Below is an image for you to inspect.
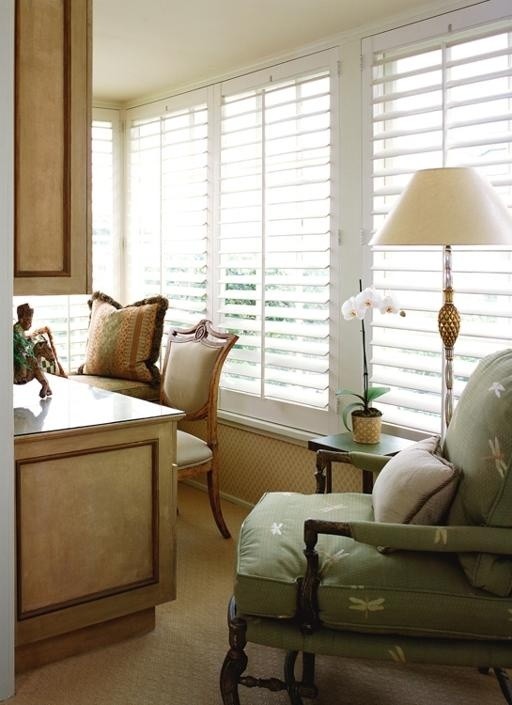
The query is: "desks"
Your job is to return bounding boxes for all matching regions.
[309,432,419,494]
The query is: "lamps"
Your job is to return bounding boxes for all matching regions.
[368,164,512,428]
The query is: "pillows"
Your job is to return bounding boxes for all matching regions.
[370,434,463,554]
[76,290,168,388]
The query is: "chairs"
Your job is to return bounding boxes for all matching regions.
[217,347,510,705]
[159,319,240,541]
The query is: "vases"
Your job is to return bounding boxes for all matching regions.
[349,411,383,445]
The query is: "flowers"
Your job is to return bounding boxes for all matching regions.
[335,277,407,436]
